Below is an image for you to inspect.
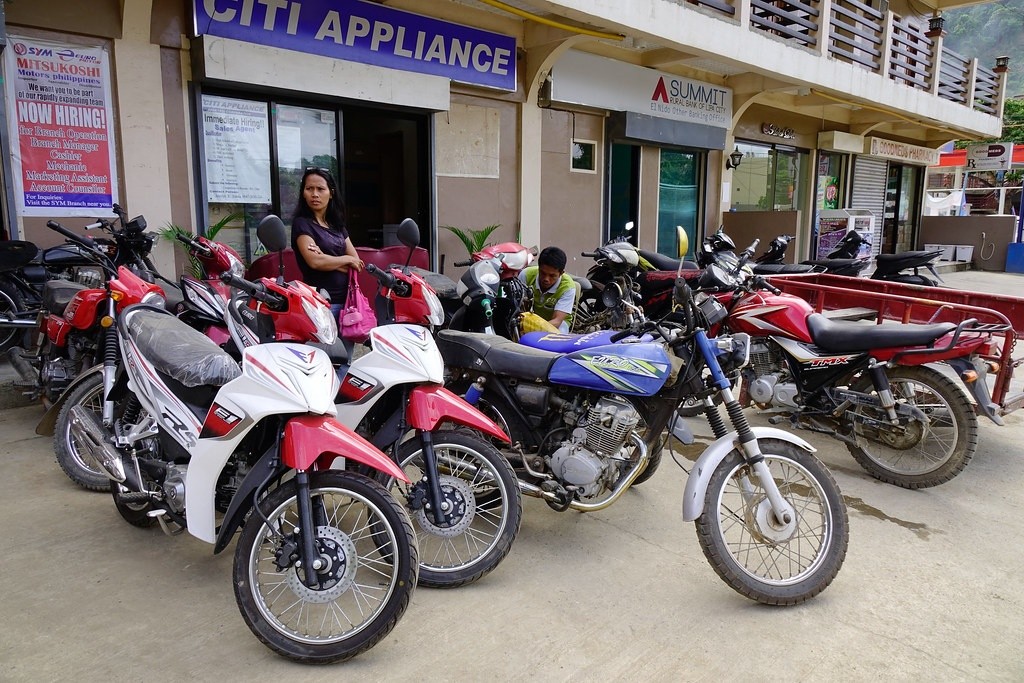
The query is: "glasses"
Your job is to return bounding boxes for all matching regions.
[305,165,332,175]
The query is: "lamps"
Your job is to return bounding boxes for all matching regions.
[726,145,744,170]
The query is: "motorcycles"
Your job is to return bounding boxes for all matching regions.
[634,228,1005,488]
[387,243,850,609]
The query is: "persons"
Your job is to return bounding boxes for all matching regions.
[514,246,576,334]
[292,167,365,400]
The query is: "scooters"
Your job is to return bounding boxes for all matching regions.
[0,202,524,666]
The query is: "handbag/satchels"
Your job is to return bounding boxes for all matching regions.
[338,266,377,342]
